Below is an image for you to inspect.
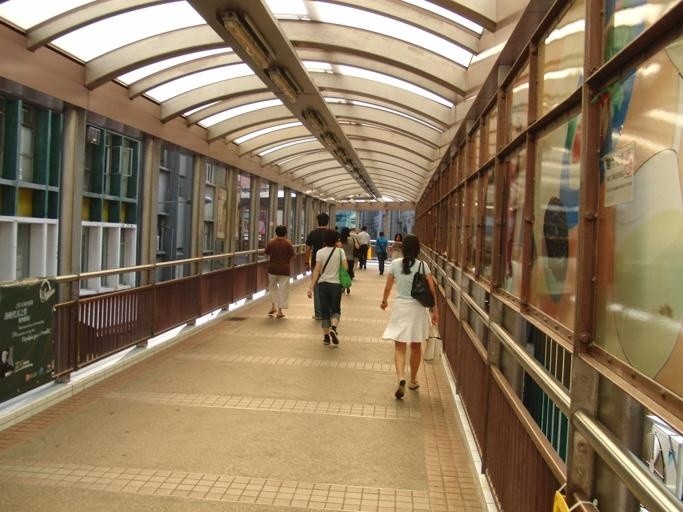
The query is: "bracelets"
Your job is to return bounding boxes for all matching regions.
[308,285,312,289]
[304,262,308,265]
[381,300,386,304]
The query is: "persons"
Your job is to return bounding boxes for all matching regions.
[379,234,439,398]
[334,226,401,294]
[265,225,295,316]
[304,212,341,321]
[306,228,348,344]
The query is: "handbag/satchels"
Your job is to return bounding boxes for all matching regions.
[410,273,436,308]
[424,337,445,366]
[384,252,388,260]
[339,268,352,288]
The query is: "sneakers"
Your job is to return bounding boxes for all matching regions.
[408,382,422,391]
[267,308,286,319]
[393,378,406,398]
[322,330,340,347]
[359,266,384,276]
[366,247,373,261]
[310,315,322,320]
[352,245,361,257]
